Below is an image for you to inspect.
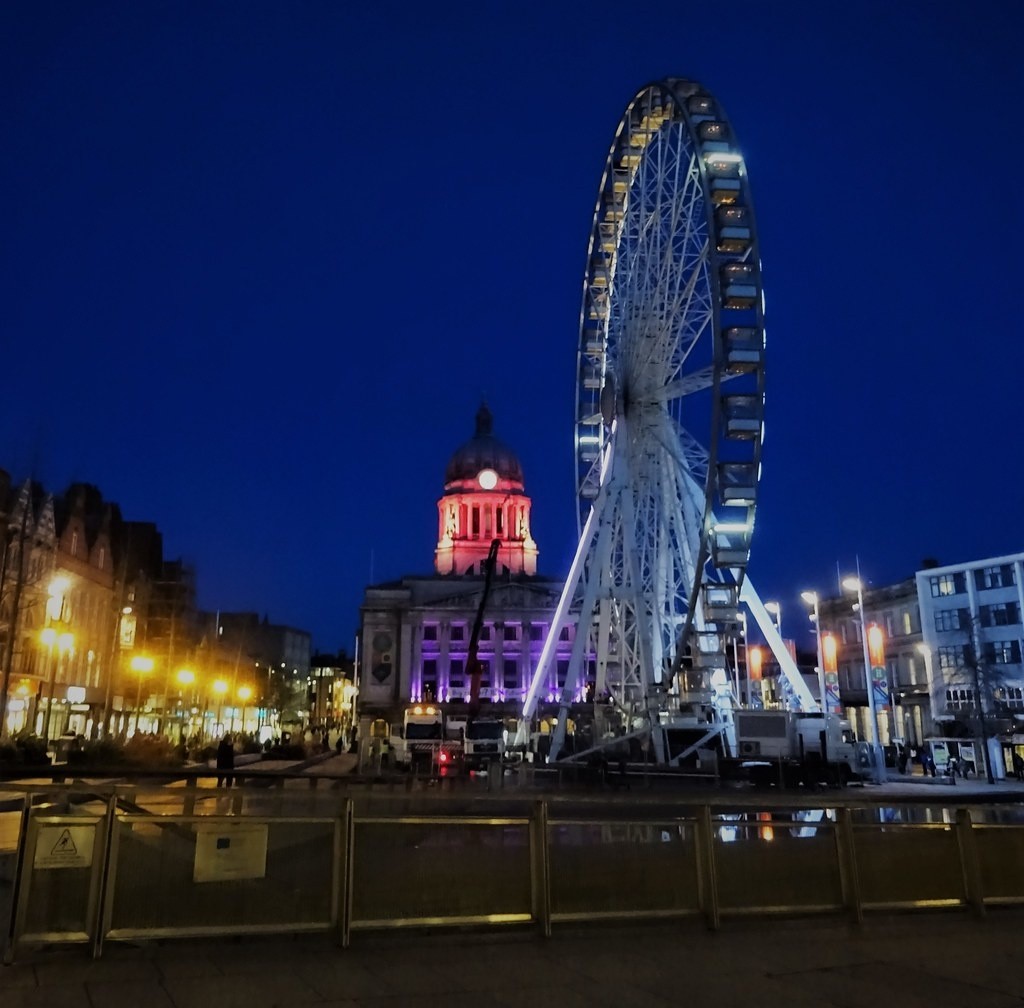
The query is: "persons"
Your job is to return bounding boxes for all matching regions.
[897,744,936,777]
[217,733,235,788]
[1013,753,1024,782]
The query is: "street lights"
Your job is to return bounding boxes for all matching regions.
[799,590,828,715]
[237,685,253,735]
[176,669,195,743]
[128,654,155,734]
[210,677,229,740]
[841,577,887,785]
[42,628,77,737]
[736,610,757,709]
[763,599,786,713]
